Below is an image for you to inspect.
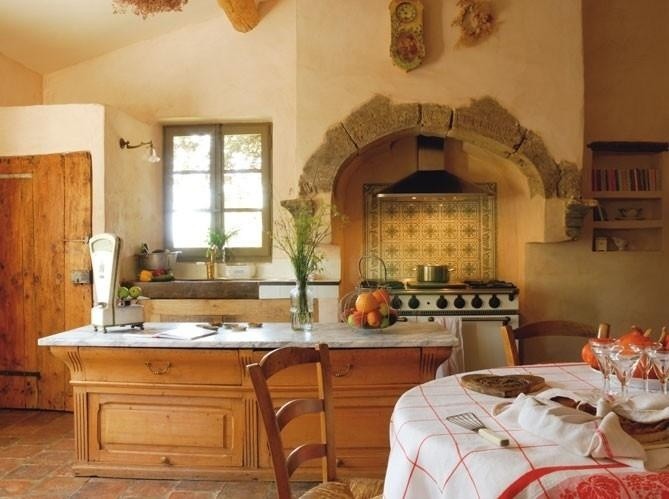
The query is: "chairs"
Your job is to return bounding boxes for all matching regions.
[499,321,611,366]
[246,342,385,499]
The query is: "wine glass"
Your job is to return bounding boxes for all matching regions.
[588,338,669,399]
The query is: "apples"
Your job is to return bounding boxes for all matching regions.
[129,285,141,297]
[118,287,128,298]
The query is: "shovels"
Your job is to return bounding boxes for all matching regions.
[445,412,509,449]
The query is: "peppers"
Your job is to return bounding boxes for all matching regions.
[139,270,153,281]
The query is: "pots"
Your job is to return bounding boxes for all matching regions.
[410,264,455,283]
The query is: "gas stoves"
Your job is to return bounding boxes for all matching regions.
[359,279,520,315]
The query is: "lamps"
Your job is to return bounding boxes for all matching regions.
[119,135,161,164]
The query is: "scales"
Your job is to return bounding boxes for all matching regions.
[87,232,149,333]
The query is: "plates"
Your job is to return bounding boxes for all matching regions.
[614,216,645,220]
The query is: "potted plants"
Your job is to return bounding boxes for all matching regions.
[267,199,345,331]
[206,226,237,263]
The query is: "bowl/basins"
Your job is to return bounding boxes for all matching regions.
[139,252,178,270]
[613,240,628,250]
[618,207,642,216]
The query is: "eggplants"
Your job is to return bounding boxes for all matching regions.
[151,267,173,276]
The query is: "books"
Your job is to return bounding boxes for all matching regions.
[151,326,216,340]
[592,168,661,192]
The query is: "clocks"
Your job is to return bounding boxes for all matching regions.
[389,0,426,73]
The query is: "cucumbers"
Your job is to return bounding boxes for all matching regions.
[155,272,176,283]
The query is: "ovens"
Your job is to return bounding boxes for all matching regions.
[455,313,520,372]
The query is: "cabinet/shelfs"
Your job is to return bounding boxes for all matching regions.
[253,346,456,482]
[585,139,666,253]
[51,347,252,480]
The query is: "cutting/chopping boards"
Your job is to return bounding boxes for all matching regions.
[461,373,545,398]
